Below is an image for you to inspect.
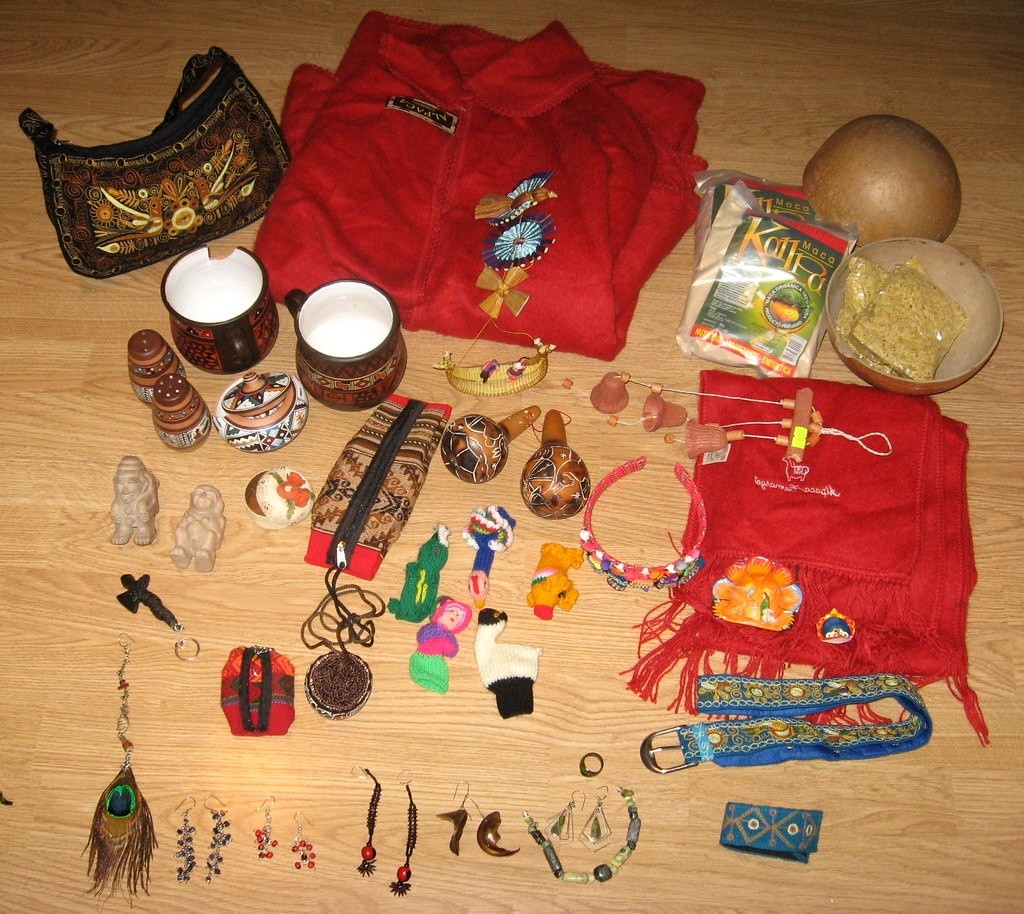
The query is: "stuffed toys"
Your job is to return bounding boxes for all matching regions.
[527,542,584,621]
[388,523,453,623]
[410,596,472,693]
[473,608,542,720]
[461,505,516,608]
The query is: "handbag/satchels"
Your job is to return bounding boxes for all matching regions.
[303,394,459,581]
[19,46,292,279]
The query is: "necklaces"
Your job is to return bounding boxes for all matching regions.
[522,787,641,883]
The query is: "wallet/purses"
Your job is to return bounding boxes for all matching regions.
[220,645,296,738]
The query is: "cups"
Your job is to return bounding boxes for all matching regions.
[161,245,279,373]
[285,278,405,412]
[244,465,315,530]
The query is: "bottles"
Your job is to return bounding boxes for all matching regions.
[128,330,186,404]
[151,376,211,450]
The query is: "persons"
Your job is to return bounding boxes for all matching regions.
[108,456,160,545]
[169,483,226,572]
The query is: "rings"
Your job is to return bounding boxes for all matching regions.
[580,753,604,778]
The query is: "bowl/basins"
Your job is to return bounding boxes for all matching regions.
[802,115,962,247]
[825,238,1003,396]
